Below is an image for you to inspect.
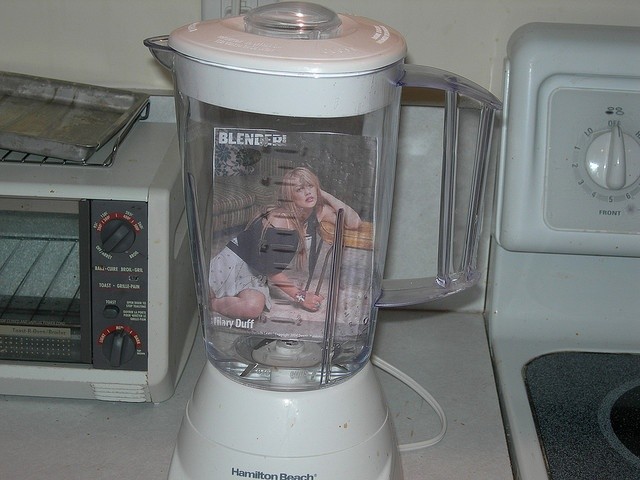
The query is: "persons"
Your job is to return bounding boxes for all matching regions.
[205,164,361,321]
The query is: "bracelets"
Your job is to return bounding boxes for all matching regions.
[295,288,307,303]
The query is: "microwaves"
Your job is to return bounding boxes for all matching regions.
[1,86,202,401]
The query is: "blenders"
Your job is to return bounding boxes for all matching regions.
[143,0,504,480]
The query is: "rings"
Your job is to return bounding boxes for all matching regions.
[317,302,320,307]
